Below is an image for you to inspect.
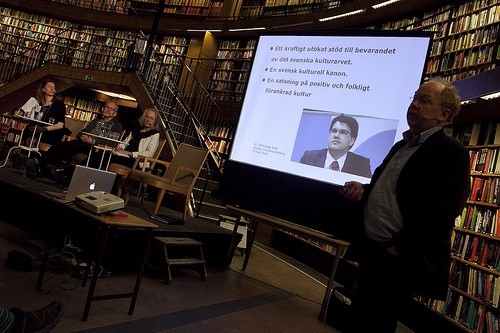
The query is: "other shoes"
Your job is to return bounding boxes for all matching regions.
[20,153,36,171]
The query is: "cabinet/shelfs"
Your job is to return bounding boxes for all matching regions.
[0,0,500,333]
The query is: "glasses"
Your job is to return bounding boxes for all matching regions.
[103,105,116,112]
[329,128,352,137]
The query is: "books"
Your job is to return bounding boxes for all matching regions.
[197,121,232,174]
[51,0,341,20]
[0,5,187,93]
[0,114,37,147]
[55,93,103,122]
[312,240,338,254]
[204,37,256,102]
[366,0,500,333]
[75,191,124,214]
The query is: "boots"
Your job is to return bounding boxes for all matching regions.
[9,300,64,333]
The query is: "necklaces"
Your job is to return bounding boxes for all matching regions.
[40,103,52,120]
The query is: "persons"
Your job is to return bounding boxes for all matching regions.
[300,115,371,178]
[0,298,65,333]
[34,101,122,178]
[19,78,66,158]
[69,108,161,178]
[340,77,471,333]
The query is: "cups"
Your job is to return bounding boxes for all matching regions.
[48,118,55,124]
[99,130,121,140]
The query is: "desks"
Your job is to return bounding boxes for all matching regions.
[37,193,159,322]
[83,132,129,171]
[0,114,54,168]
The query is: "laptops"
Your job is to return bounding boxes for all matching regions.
[44,165,116,201]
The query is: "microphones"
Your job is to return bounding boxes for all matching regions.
[423,115,438,120]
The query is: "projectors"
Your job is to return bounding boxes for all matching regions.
[74,191,125,213]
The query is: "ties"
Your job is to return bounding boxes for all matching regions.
[331,161,339,171]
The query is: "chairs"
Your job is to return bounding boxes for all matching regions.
[108,139,166,197]
[124,143,210,221]
